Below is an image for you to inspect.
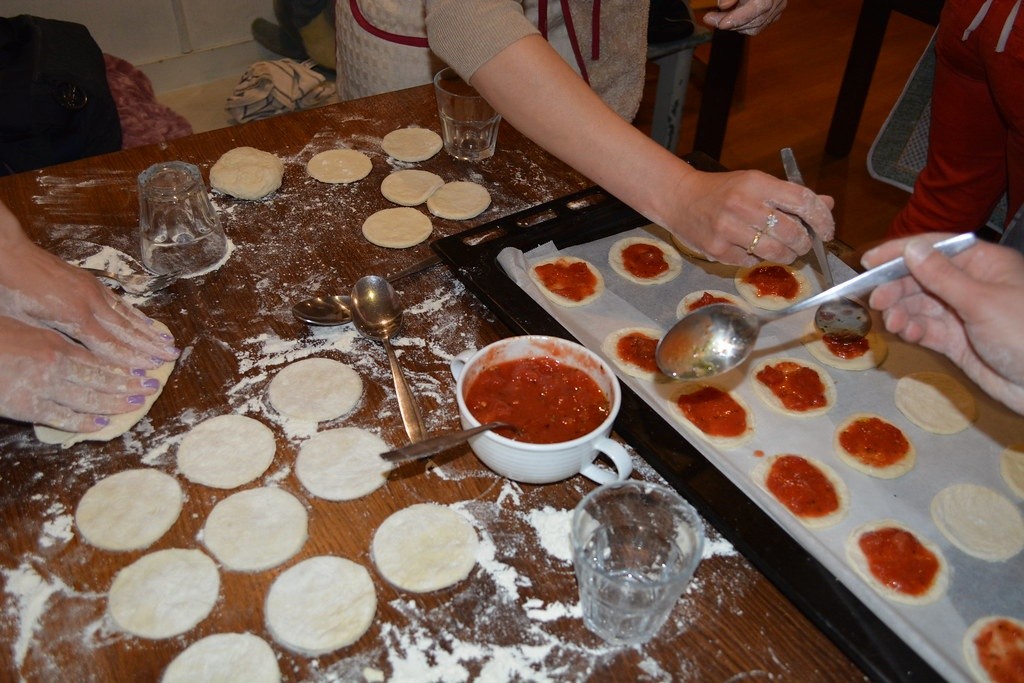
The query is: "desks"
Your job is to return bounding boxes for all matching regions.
[0,79,1024,683]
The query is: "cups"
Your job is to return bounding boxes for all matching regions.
[448,335,632,489]
[568,479,705,646]
[137,162,229,274]
[433,66,501,162]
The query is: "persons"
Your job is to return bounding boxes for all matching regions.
[335,0,835,268]
[884,0,1024,240]
[860,233,1024,416]
[0,201,182,434]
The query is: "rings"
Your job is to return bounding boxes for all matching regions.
[762,209,778,234]
[747,232,762,254]
[111,300,122,310]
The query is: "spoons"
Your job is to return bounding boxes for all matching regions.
[291,255,445,328]
[780,147,872,344]
[349,275,428,445]
[655,231,976,380]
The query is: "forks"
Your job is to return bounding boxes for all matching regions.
[85,267,185,296]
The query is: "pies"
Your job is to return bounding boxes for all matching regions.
[33,128,1024,683]
